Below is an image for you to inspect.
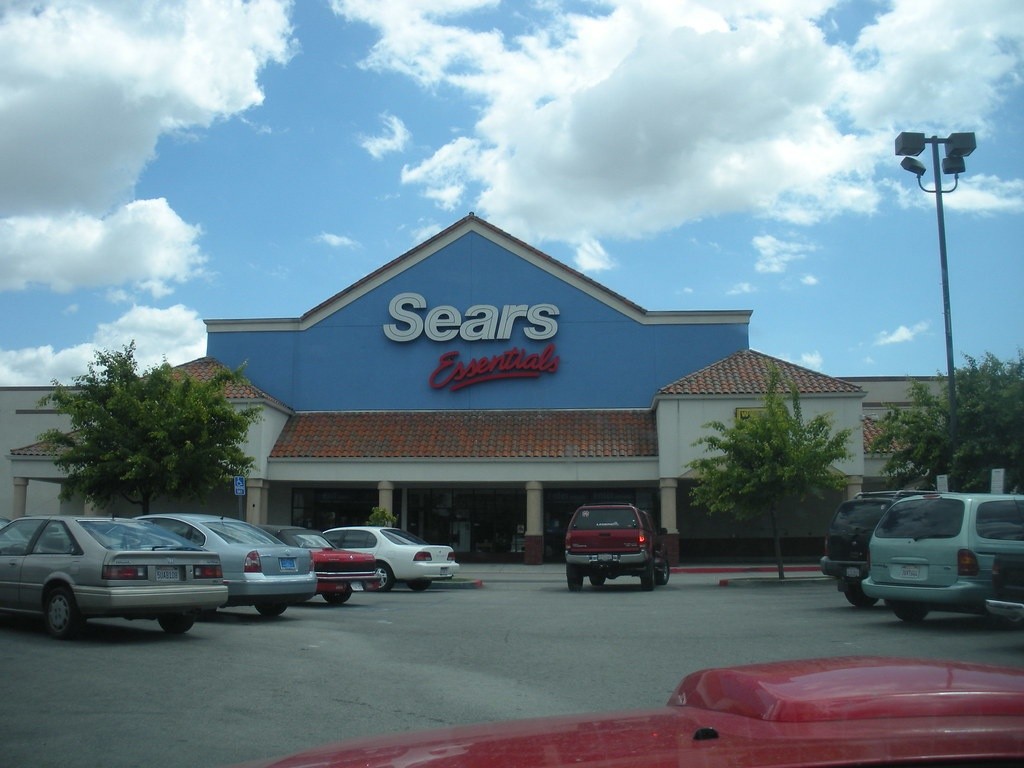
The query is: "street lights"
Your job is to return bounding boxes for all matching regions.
[895,131,979,453]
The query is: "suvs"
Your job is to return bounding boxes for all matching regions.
[820,491,942,606]
[564,504,670,590]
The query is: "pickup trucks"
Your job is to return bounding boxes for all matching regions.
[991,552,1024,601]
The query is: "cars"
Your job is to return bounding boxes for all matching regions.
[252,524,380,604]
[0,514,228,634]
[229,656,1024,768]
[0,515,27,549]
[130,513,318,617]
[321,526,456,593]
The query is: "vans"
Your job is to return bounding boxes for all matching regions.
[861,492,1024,621]
